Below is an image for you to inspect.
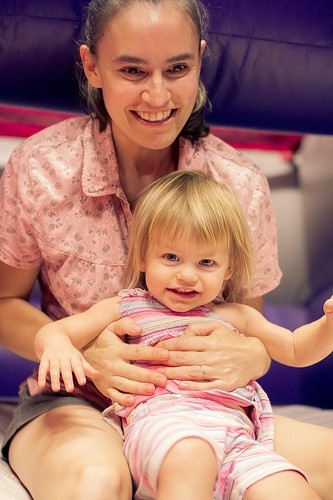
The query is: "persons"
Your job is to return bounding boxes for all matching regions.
[33,168,333,500]
[0,0,332,500]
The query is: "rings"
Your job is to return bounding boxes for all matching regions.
[201,366,206,379]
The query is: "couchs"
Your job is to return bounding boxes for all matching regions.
[1,101,333,498]
